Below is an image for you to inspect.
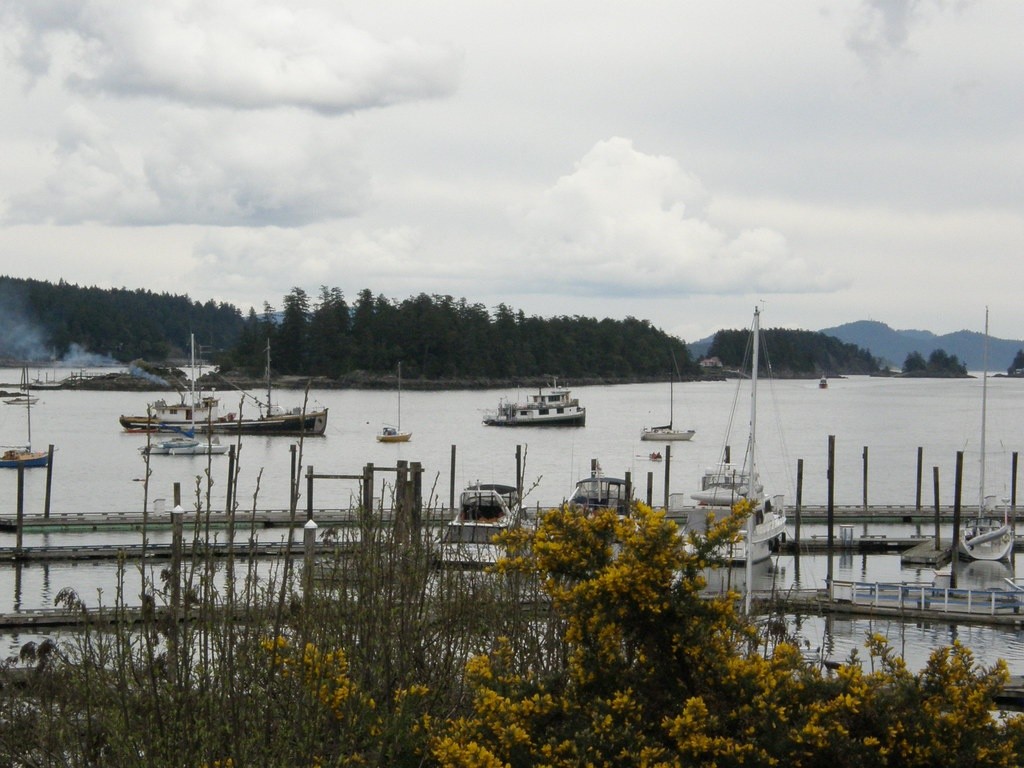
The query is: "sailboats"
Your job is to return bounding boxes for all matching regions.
[682,305,790,568]
[118,332,328,437]
[376,362,412,442]
[639,347,697,443]
[0,364,49,468]
[959,303,1015,561]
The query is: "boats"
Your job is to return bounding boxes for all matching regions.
[566,475,635,517]
[819,377,828,388]
[479,372,587,428]
[3,397,37,405]
[21,380,62,390]
[445,483,520,545]
[139,438,229,456]
